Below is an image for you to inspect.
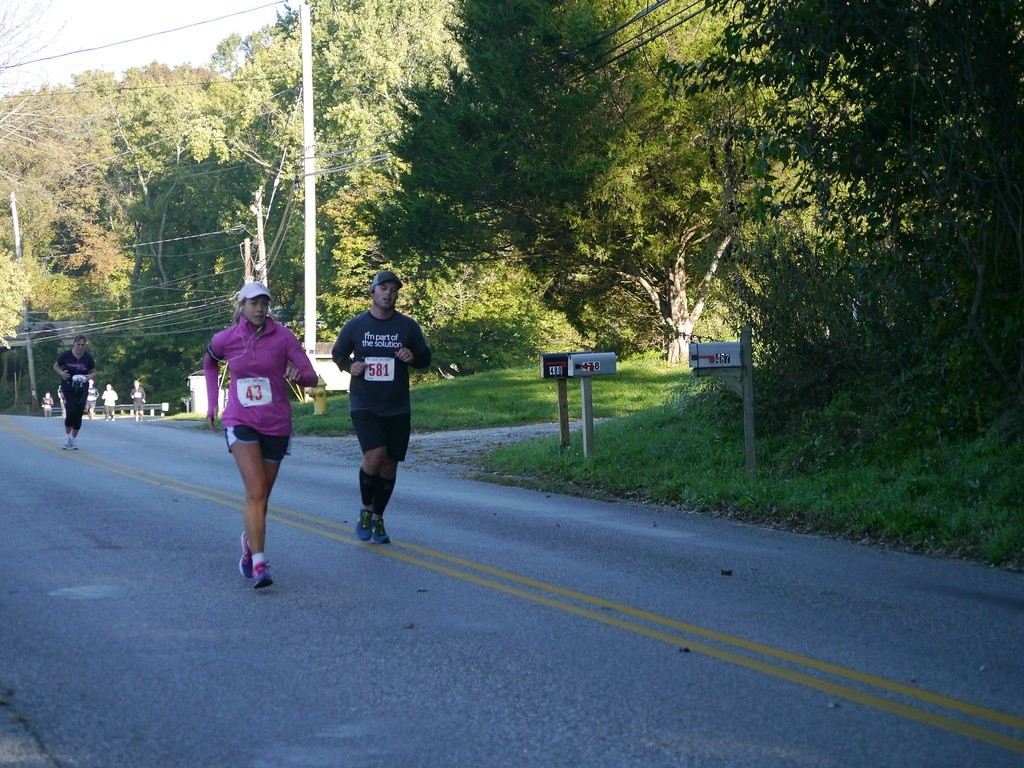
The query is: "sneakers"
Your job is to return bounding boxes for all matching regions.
[357,508,373,540]
[371,518,397,541]
[239,530,252,578]
[252,560,274,588]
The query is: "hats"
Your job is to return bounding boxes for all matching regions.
[239,282,273,302]
[372,271,404,289]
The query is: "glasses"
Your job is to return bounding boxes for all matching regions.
[73,344,85,346]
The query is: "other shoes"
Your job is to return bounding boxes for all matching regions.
[62,438,78,449]
[105,419,108,421]
[112,419,115,421]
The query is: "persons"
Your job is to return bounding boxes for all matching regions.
[84,380,99,419]
[41,392,54,417]
[102,384,118,421]
[58,385,66,418]
[53,335,96,449]
[331,271,432,544]
[130,380,146,422]
[203,282,319,589]
